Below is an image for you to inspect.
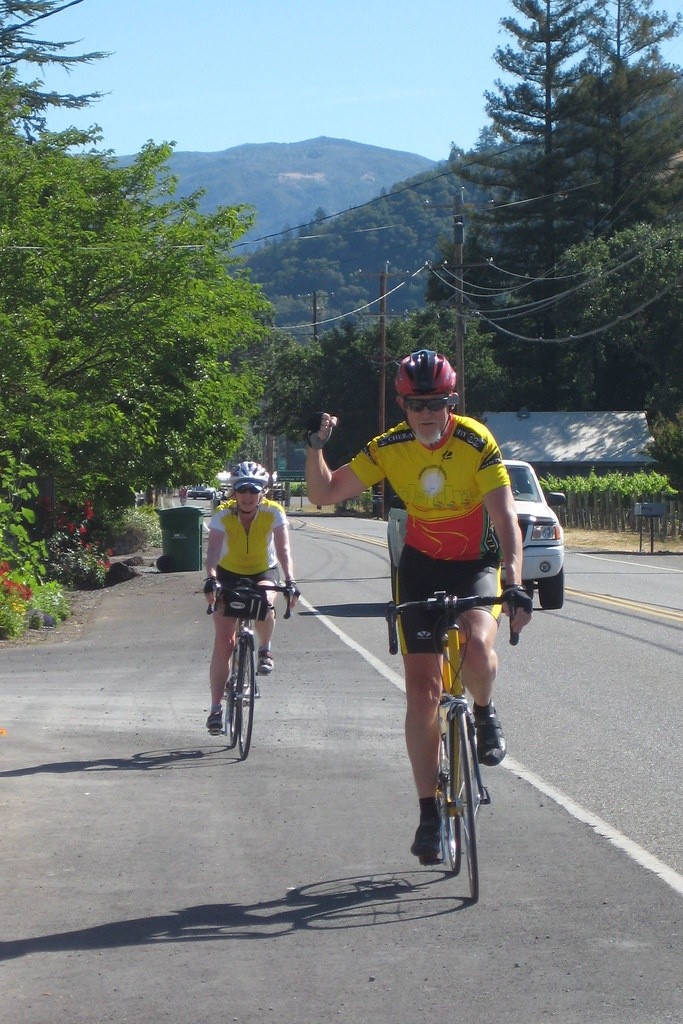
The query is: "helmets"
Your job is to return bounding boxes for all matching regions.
[229,461,269,488]
[395,350,456,396]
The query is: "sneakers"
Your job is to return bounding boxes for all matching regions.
[257,649,274,674]
[472,700,507,767]
[410,812,443,857]
[206,712,224,729]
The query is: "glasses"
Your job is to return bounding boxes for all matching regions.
[237,486,261,494]
[403,398,450,413]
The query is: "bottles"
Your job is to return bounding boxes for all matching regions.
[438,696,448,758]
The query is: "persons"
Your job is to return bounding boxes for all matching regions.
[203,459,302,736]
[179,487,188,498]
[305,350,535,864]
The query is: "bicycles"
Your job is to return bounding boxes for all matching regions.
[206,577,292,760]
[386,590,519,903]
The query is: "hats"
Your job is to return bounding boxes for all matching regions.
[232,464,265,491]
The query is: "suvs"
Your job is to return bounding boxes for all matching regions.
[387,459,568,610]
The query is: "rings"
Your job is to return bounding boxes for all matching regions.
[323,425,328,430]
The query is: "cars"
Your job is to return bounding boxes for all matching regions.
[177,484,230,510]
[263,483,287,501]
